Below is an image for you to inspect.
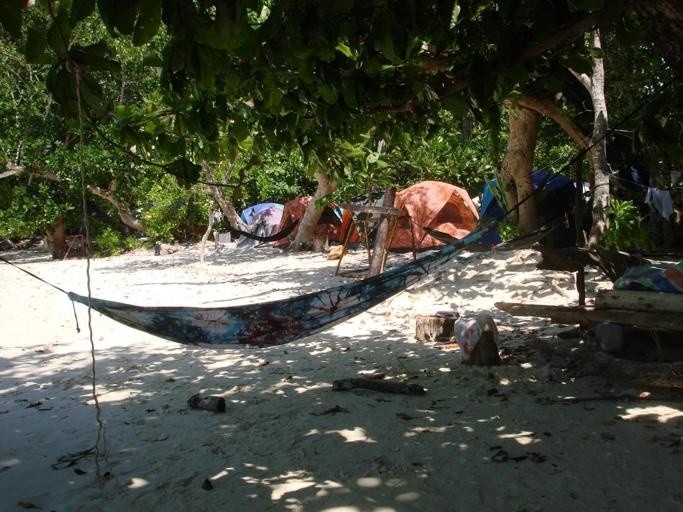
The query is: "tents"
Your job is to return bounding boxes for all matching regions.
[243,203,278,226]
[386,180,479,251]
[274,196,361,247]
[479,170,575,241]
[237,207,285,248]
